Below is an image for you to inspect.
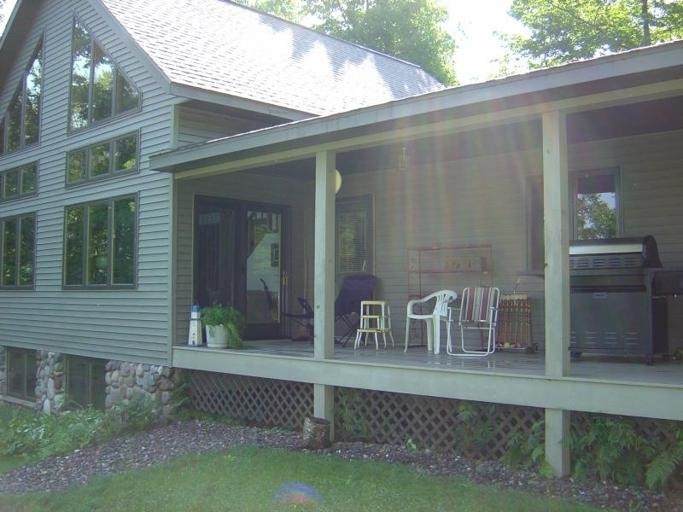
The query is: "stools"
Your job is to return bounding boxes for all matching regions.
[360,300,391,329]
[354,328,395,350]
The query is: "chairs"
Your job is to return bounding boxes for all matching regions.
[285,273,377,347]
[403,289,458,355]
[447,287,500,357]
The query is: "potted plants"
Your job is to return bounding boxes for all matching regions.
[200,305,246,348]
